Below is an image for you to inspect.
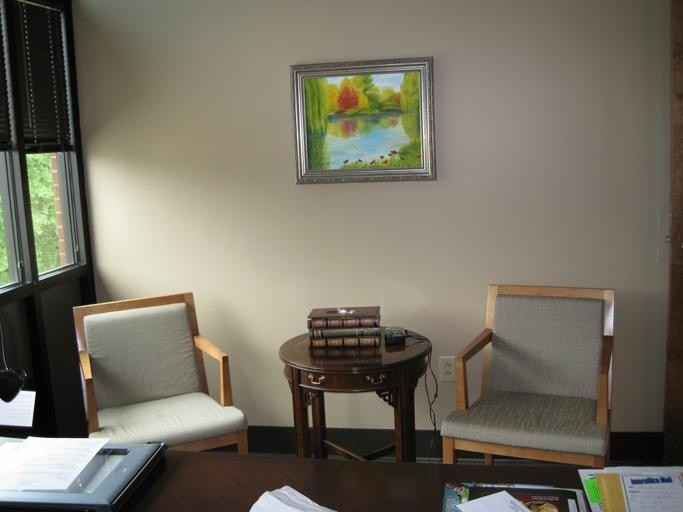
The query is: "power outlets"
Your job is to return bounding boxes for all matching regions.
[438,355,454,382]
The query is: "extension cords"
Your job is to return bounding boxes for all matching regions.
[385,326,409,344]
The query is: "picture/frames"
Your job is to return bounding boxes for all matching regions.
[289,55,437,185]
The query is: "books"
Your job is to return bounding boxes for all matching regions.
[308,305,382,347]
[312,347,383,369]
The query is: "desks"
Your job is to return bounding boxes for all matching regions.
[0,452,592,511]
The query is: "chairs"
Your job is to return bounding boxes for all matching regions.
[72,291,247,453]
[439,283,616,468]
